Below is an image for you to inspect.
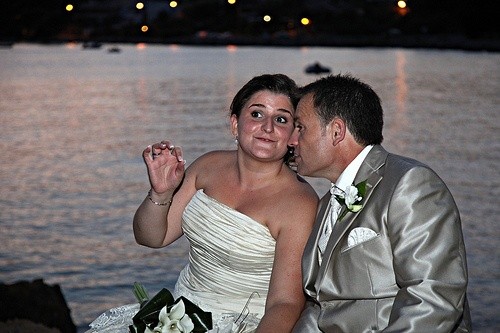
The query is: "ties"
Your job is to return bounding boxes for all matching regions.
[312,185,347,273]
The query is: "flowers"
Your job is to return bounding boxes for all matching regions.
[335,179,367,221]
[130,281,213,333]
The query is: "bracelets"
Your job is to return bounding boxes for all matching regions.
[148,188,172,206]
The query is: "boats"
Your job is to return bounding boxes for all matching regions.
[305,62,332,75]
[81,42,124,54]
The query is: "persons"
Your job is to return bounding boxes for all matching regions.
[84,72,320,333]
[287,72,475,333]
